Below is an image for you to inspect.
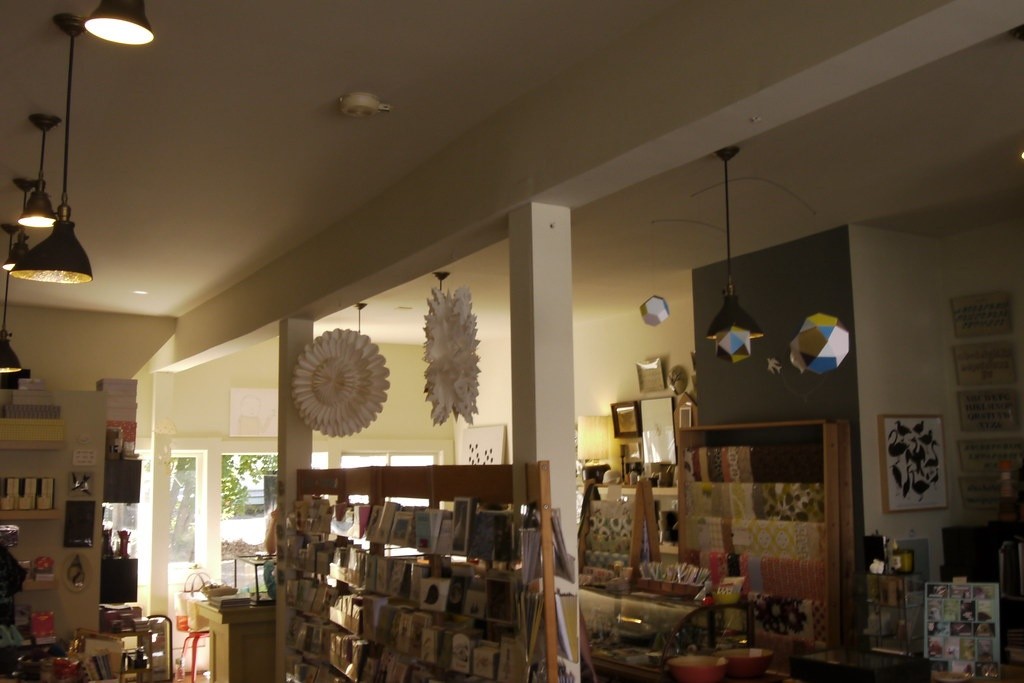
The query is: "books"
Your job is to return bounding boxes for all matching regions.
[133,616,150,632]
[210,595,252,607]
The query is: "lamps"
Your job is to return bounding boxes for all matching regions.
[705,146,764,364]
[0,0,156,389]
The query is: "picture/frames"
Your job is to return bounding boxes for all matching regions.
[610,401,642,438]
[877,413,951,512]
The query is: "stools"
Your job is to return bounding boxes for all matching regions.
[177,619,212,683]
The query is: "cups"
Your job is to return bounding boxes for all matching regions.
[893,550,915,574]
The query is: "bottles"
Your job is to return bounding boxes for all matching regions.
[995,457,1016,522]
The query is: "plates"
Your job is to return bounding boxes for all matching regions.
[61,552,94,590]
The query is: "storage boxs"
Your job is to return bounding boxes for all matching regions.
[96,379,138,455]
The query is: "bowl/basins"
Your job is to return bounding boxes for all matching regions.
[666,655,728,683]
[713,648,774,677]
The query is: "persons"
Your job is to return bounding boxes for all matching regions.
[264,506,278,601]
